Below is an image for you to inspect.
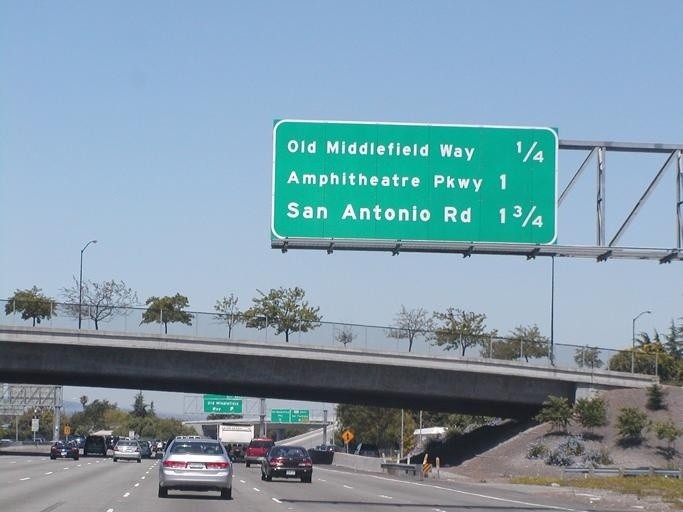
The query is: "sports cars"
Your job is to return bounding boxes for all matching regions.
[49,440,78,460]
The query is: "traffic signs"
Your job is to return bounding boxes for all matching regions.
[271,118,563,246]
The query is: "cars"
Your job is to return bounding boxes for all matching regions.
[71,436,276,468]
[158,434,233,500]
[307,444,339,464]
[260,444,312,483]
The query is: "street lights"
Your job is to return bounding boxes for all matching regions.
[630,309,653,374]
[78,238,97,329]
